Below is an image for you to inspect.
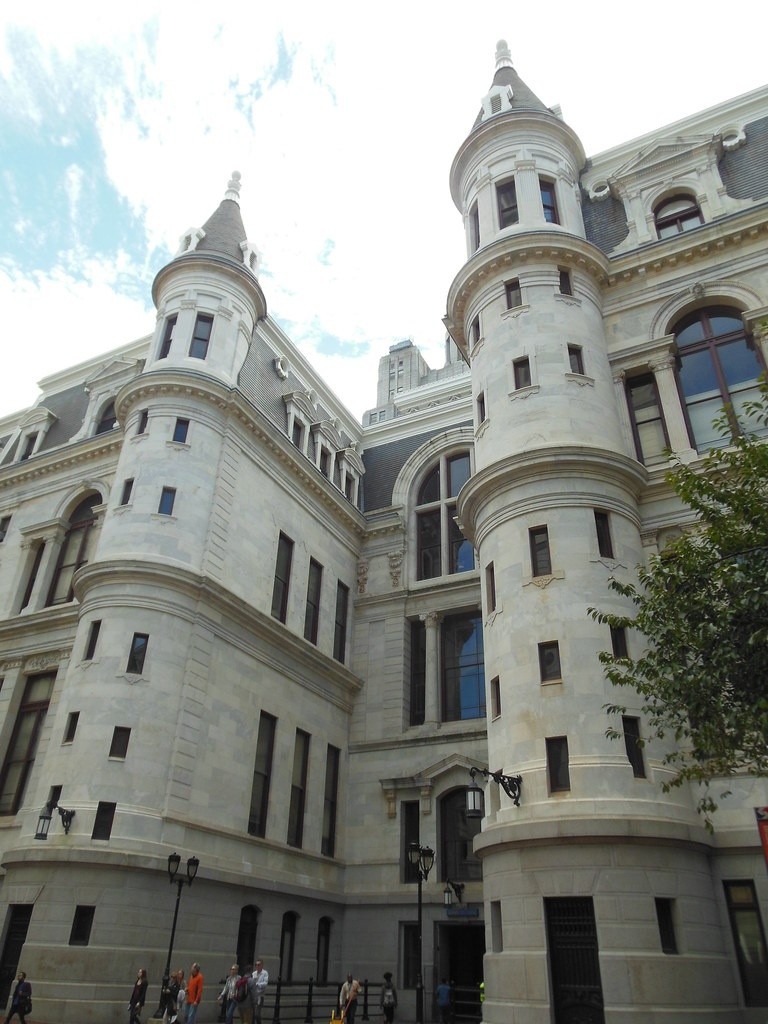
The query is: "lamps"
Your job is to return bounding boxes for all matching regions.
[33,800,74,840]
[444,879,464,908]
[468,766,522,807]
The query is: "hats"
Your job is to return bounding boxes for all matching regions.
[383,972,393,978]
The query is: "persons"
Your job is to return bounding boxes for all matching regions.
[340,972,361,1024]
[3,972,32,1024]
[480,982,485,1003]
[127,969,148,1024]
[380,972,398,1024]
[163,963,204,1024]
[218,959,269,1024]
[435,977,454,1024]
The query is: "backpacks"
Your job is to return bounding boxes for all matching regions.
[234,976,249,1002]
[382,985,394,1007]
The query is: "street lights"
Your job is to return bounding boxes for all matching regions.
[148,850,201,1024]
[407,842,436,1024]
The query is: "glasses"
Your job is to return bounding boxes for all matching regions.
[171,976,176,979]
[230,969,237,970]
[255,964,262,966]
